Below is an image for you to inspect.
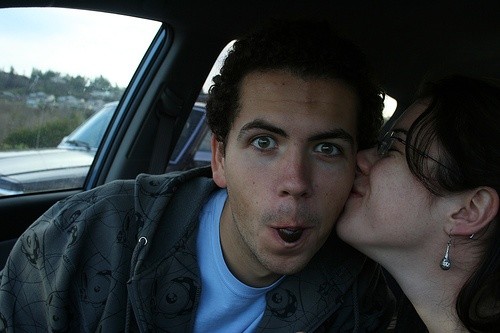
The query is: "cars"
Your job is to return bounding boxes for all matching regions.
[0,98,212,198]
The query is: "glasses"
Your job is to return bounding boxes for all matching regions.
[376,126,473,186]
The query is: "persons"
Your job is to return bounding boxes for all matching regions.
[1,11,431,333]
[336,66,500,333]
